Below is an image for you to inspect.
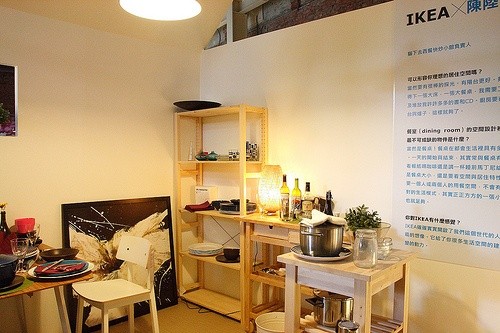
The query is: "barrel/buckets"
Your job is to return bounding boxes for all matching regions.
[254,311,306,333]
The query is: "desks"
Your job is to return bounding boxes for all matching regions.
[277,245,417,333]
[0,244,93,333]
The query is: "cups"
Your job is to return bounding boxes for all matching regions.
[15,218,35,233]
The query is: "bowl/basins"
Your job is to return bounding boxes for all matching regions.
[211,199,256,211]
[223,248,239,260]
[41,249,79,262]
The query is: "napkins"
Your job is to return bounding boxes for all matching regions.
[34,262,86,274]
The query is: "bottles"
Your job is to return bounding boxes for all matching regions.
[290,178,302,221]
[279,174,290,221]
[301,182,314,218]
[195,141,258,161]
[353,229,376,269]
[0,211,16,253]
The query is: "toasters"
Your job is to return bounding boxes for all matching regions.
[195,185,218,205]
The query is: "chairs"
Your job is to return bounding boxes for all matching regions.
[72,235,159,333]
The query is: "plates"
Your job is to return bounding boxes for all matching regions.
[216,255,240,262]
[291,245,352,262]
[174,100,222,111]
[27,260,95,280]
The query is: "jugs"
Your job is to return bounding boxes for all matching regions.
[338,317,360,333]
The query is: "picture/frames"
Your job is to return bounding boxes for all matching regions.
[0,64,19,137]
[61,196,179,333]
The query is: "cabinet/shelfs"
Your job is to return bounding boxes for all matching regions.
[234,213,305,333]
[173,104,267,321]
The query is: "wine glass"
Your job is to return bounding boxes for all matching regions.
[256,192,270,219]
[10,225,41,274]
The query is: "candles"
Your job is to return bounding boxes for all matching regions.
[15,218,35,233]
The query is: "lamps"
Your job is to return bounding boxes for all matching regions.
[258,165,284,216]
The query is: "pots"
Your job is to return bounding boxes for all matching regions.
[299,221,344,255]
[0,253,19,288]
[305,288,354,328]
